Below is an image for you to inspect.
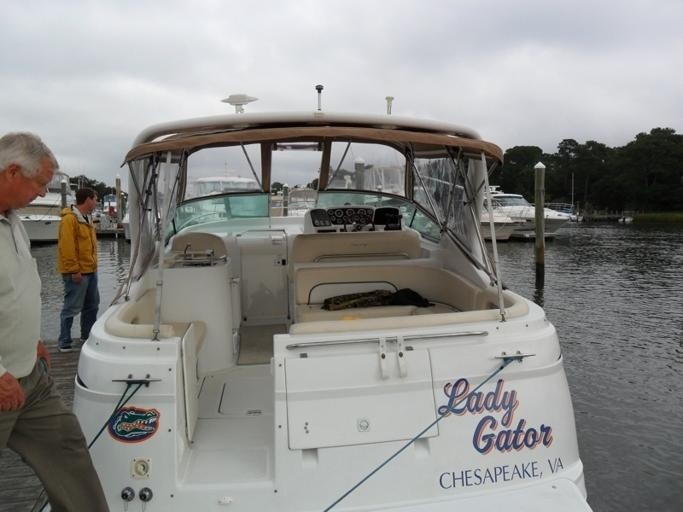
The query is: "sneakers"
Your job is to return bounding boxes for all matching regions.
[58,336,87,353]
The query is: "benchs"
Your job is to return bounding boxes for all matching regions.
[277,228,527,333]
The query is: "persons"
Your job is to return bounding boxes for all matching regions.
[0,132,108,512]
[58,188,102,353]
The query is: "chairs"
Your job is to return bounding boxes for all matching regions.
[170,231,227,262]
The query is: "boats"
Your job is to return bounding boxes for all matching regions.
[72,86,590,512]
[62,163,584,246]
[16,169,76,242]
